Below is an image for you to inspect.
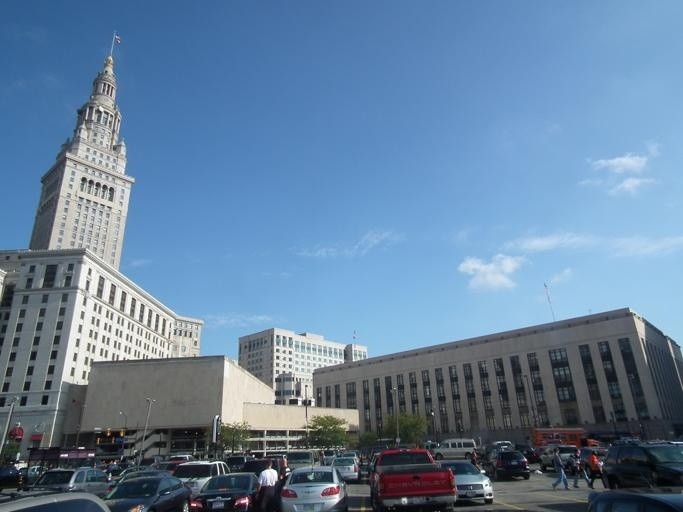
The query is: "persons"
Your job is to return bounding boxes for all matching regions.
[589,450,606,489]
[255,460,277,512]
[278,458,292,485]
[470,449,481,474]
[550,448,571,490]
[568,449,590,488]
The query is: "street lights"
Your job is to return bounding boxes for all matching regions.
[392,387,400,446]
[429,411,437,448]
[117,410,127,461]
[303,382,308,426]
[137,396,158,468]
[0,395,20,452]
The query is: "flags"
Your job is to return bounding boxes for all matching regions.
[114,33,120,44]
[351,329,358,340]
